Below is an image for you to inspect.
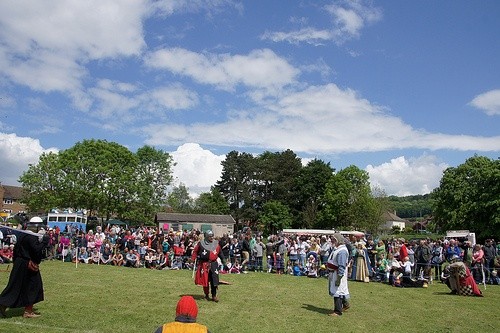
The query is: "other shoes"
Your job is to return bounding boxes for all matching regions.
[204,295,209,301]
[212,296,219,302]
[0,305,8,317]
[23,311,41,317]
[342,303,351,311]
[328,311,342,316]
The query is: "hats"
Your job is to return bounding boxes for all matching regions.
[175,295,198,319]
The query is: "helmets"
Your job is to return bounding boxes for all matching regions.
[27,216,46,226]
[204,229,214,237]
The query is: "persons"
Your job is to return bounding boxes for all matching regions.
[155,294,211,333]
[0,215,44,317]
[189,229,228,301]
[0,225,203,270]
[328,233,351,315]
[216,226,500,295]
